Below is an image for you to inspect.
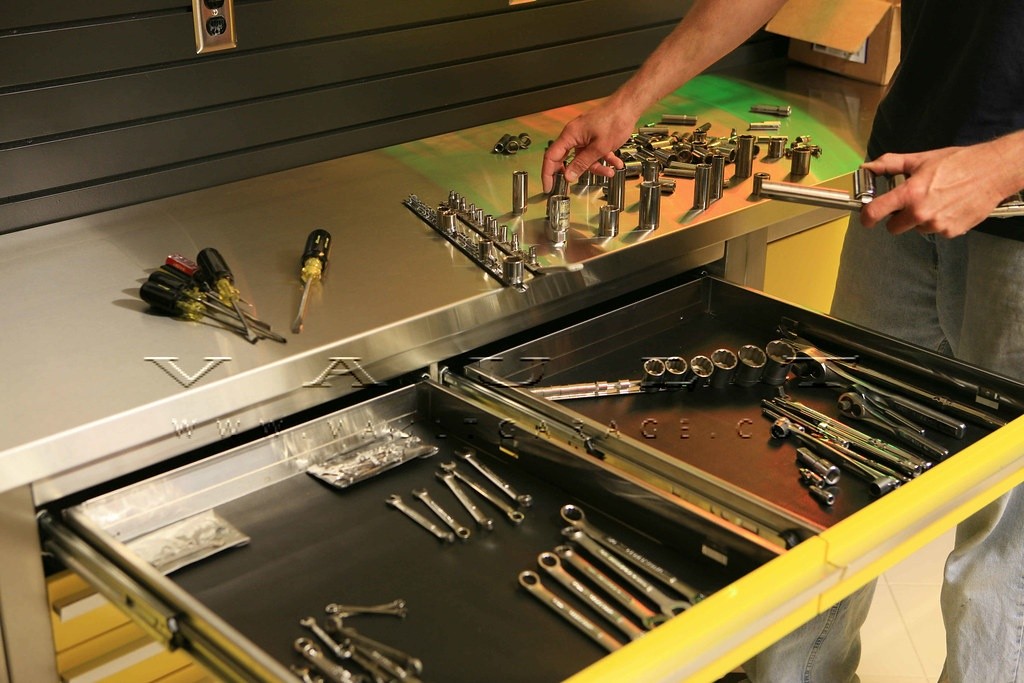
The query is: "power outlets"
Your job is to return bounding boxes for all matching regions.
[191,0,237,55]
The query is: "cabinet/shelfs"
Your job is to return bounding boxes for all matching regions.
[0,75,1024,683]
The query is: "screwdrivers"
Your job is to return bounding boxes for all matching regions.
[291,229,333,335]
[139,245,287,344]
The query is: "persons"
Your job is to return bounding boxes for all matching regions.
[541,0,1024,683]
